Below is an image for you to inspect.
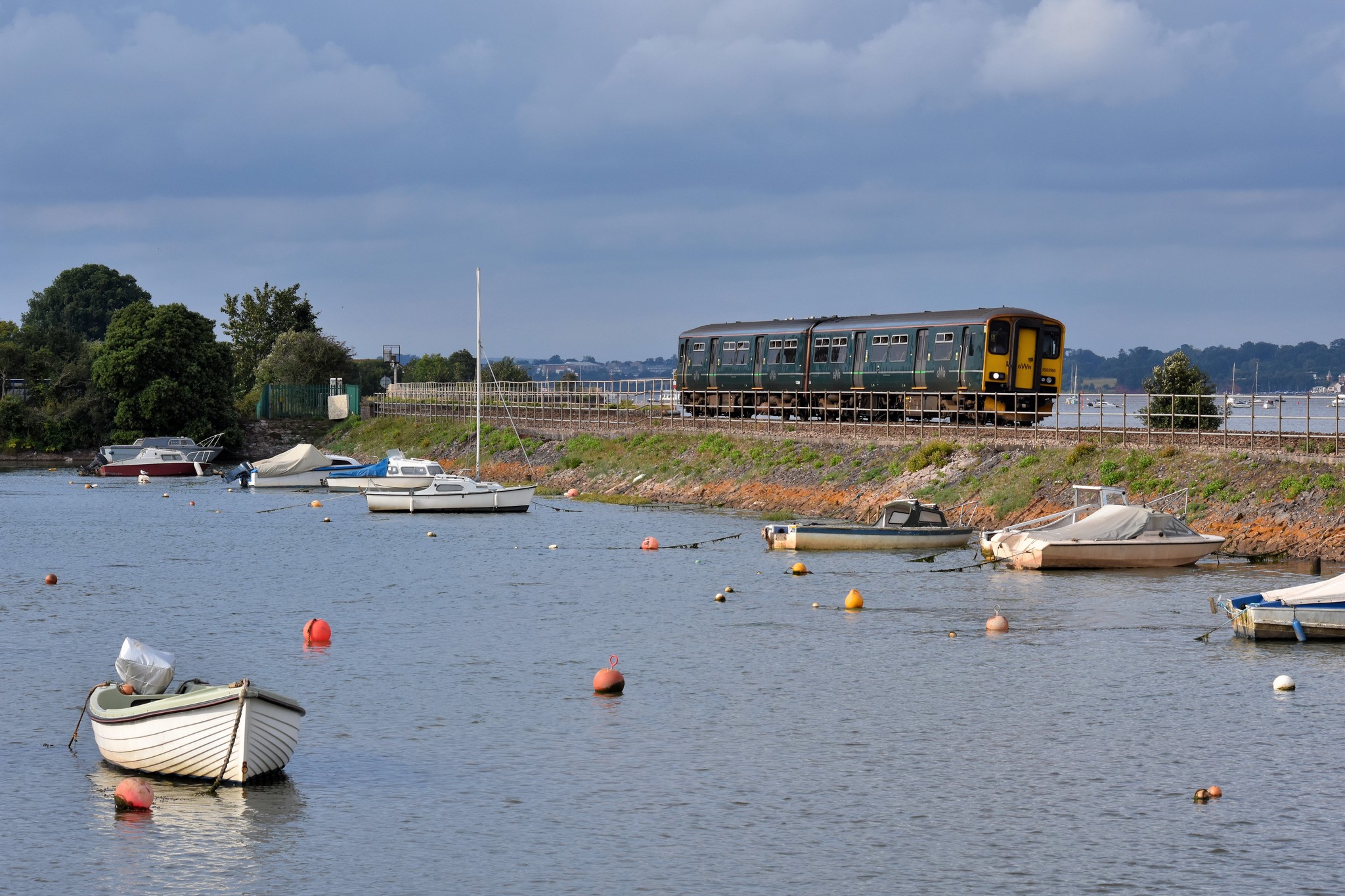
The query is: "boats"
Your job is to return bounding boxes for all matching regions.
[762,492,981,552]
[1227,572,1345,641]
[85,636,307,785]
[979,485,1226,571]
[1086,387,1116,408]
[221,443,379,488]
[77,432,229,476]
[326,449,473,493]
[1331,394,1345,407]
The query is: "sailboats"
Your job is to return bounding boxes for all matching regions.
[366,267,539,512]
[1066,363,1081,404]
[1225,361,1277,409]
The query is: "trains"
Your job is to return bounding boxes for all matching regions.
[673,305,1065,426]
